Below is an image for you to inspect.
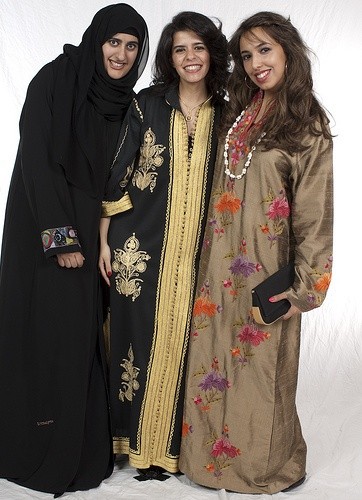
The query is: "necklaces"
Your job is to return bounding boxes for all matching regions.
[177,92,211,122]
[221,103,270,181]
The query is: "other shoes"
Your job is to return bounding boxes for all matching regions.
[134,466,170,483]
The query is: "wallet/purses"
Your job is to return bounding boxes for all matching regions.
[250,263,297,326]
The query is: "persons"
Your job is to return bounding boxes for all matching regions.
[1,1,153,498]
[99,11,239,485]
[177,10,338,496]
